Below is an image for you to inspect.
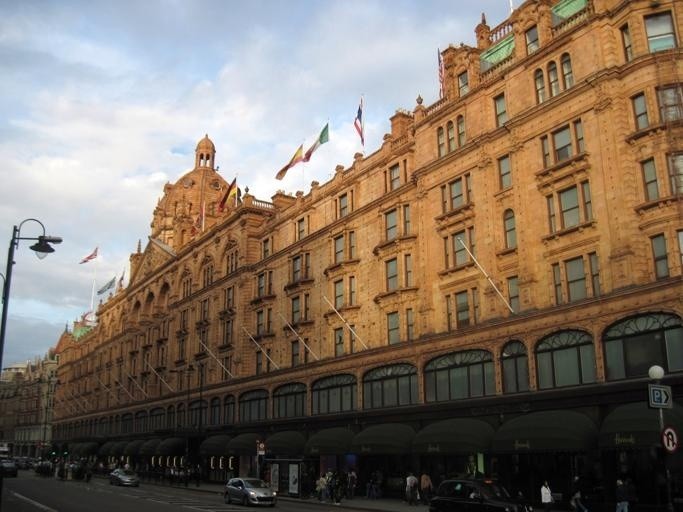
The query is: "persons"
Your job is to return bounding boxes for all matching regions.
[299,462,382,506]
[615,479,629,512]
[420,469,433,506]
[95,461,203,487]
[540,479,552,511]
[405,470,420,507]
[567,475,587,511]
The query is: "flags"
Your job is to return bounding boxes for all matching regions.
[78,258,88,265]
[302,122,329,162]
[95,276,116,296]
[216,176,236,214]
[190,204,202,238]
[84,246,97,259]
[351,95,365,147]
[274,143,303,181]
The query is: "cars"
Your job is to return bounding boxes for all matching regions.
[222,477,277,507]
[0,443,139,487]
[427,478,533,512]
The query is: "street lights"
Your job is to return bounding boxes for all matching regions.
[647,364,672,503]
[0,219,62,381]
